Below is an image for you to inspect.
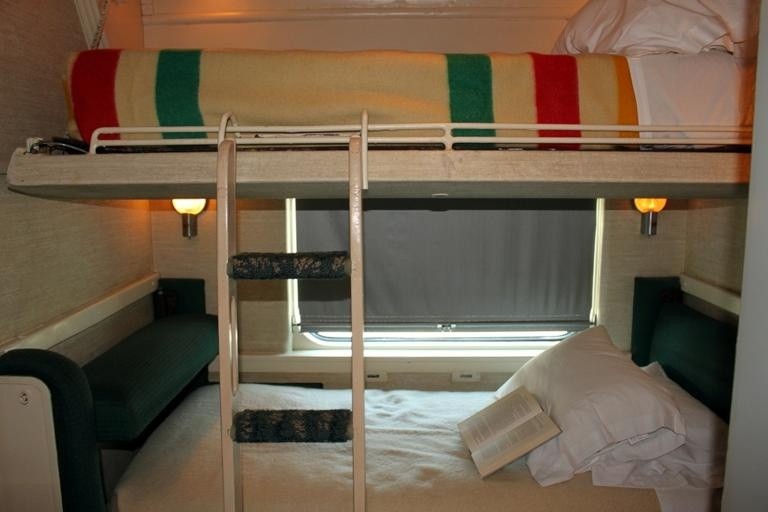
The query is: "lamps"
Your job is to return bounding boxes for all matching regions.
[632,198,668,234]
[172,198,207,238]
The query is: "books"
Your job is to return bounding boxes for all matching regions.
[458,386,563,479]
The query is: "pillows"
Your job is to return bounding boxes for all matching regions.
[491,324,686,489]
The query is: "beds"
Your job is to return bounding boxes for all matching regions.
[1,0,762,512]
